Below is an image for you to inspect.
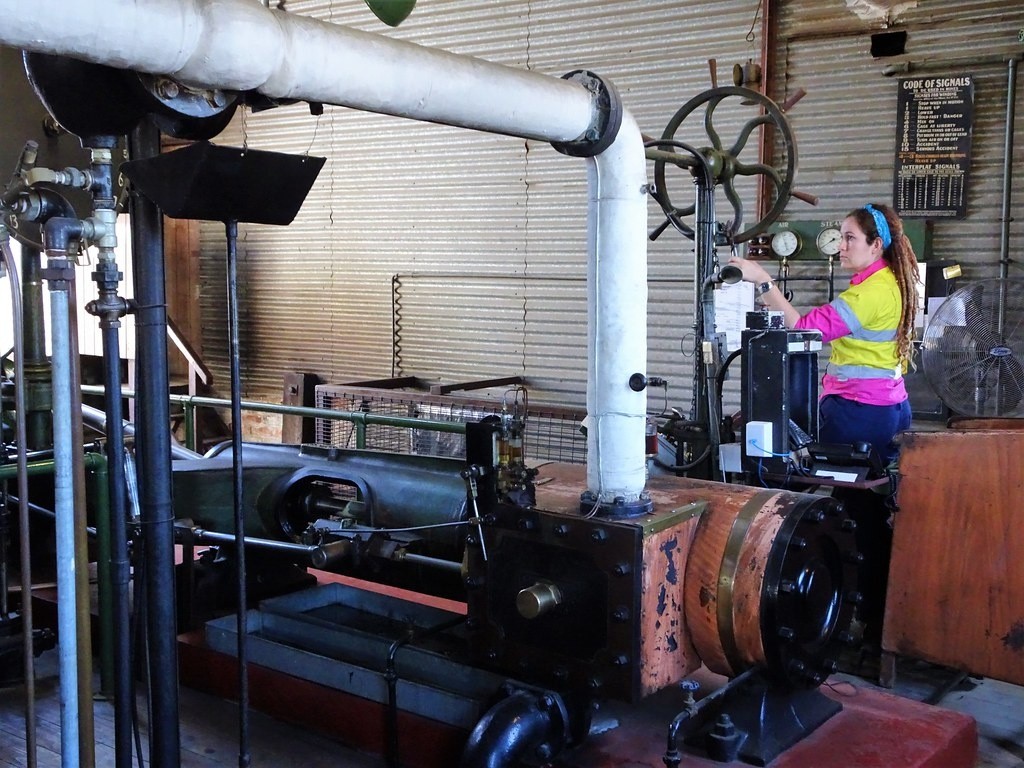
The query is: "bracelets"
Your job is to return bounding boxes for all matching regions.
[757,281,775,295]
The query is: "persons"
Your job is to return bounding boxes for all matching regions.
[728,203,922,468]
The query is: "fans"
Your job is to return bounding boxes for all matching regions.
[921,278,1024,418]
